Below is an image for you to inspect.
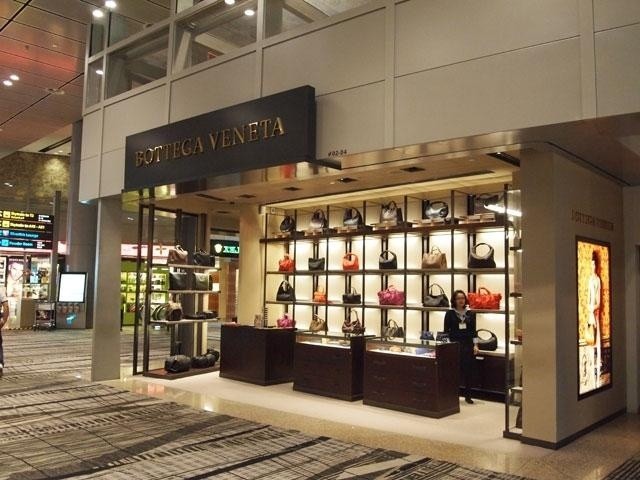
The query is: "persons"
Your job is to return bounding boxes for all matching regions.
[586,251,603,390]
[0,287,11,377]
[443,289,480,404]
[119,293,126,332]
[6,261,25,285]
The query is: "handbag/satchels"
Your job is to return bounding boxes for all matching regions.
[276,200,502,351]
[162,244,218,321]
[192,353,215,368]
[165,355,190,373]
[208,348,219,361]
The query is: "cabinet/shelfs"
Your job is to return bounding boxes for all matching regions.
[145,264,226,379]
[260,204,514,402]
[363,336,462,417]
[501,181,521,441]
[219,324,297,387]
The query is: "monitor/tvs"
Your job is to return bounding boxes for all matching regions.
[58,273,86,303]
